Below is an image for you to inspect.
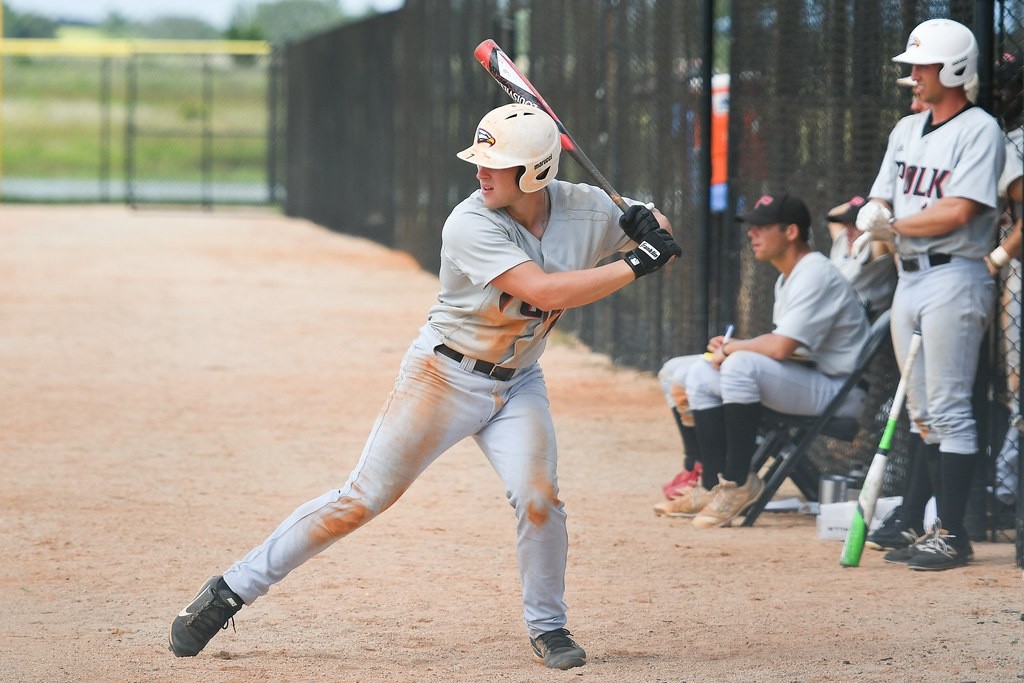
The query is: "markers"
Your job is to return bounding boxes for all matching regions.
[724,325,734,345]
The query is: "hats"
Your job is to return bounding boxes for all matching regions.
[826,197,869,226]
[732,190,814,235]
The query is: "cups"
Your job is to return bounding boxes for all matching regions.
[818,474,848,505]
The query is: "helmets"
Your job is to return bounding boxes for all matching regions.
[456,102,562,192]
[892,18,979,87]
[896,72,979,106]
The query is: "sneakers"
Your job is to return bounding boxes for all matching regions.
[691,472,765,530]
[652,472,711,518]
[864,507,926,552]
[167,575,245,658]
[908,517,967,569]
[662,461,703,501]
[529,628,586,670]
[882,517,942,564]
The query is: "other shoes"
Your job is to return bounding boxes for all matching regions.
[985,492,1017,530]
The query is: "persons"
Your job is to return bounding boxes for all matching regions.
[856,18,1005,569]
[171,103,682,669]
[867,71,983,551]
[655,189,871,529]
[661,195,897,500]
[985,124,1024,530]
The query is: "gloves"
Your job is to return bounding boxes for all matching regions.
[622,227,684,279]
[851,221,899,260]
[855,200,891,231]
[619,205,660,244]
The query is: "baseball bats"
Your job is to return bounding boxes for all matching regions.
[472,38,680,265]
[838,328,924,569]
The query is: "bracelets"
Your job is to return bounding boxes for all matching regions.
[989,245,1011,269]
[722,342,729,356]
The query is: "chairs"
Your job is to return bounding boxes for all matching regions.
[739,308,891,527]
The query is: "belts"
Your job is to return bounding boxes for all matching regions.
[900,252,951,272]
[434,344,516,381]
